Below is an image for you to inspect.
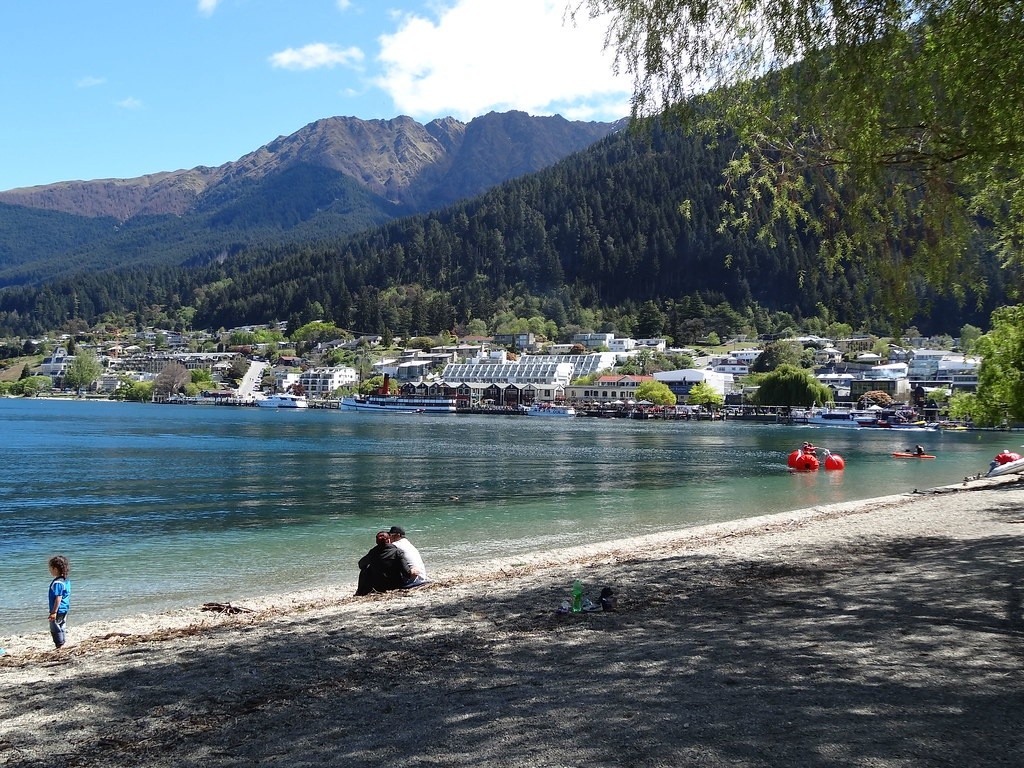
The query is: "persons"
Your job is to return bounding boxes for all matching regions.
[353,526,425,597]
[802,442,823,465]
[915,445,926,456]
[48,556,71,651]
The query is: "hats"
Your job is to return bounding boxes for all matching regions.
[389,527,405,535]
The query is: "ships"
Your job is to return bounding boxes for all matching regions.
[339,395,458,414]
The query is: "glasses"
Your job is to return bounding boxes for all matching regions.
[389,533,398,537]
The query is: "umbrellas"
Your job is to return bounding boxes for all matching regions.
[594,400,653,409]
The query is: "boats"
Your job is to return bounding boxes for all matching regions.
[528,404,577,418]
[877,418,927,427]
[258,394,309,408]
[851,416,880,428]
[807,411,860,429]
[890,424,940,429]
[893,451,936,459]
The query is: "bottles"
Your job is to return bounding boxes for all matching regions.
[561,600,570,613]
[572,579,583,613]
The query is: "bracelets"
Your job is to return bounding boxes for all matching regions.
[51,611,57,614]
